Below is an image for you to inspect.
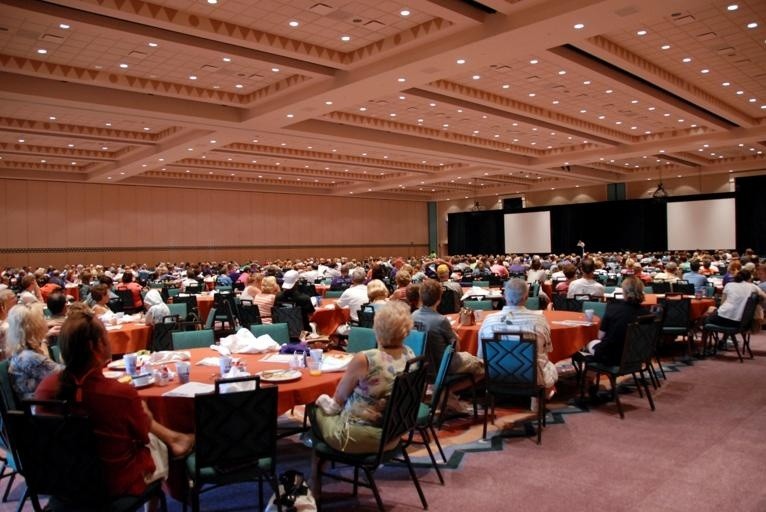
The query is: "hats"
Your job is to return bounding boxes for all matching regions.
[281,270,300,290]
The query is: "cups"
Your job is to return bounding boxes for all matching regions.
[585,309,594,322]
[111,311,125,326]
[124,354,138,375]
[177,361,191,382]
[308,363,320,375]
[309,348,322,368]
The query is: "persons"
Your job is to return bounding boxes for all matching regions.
[1,249,765,510]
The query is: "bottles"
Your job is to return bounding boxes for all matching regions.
[457,305,484,327]
[153,363,174,385]
[220,357,247,376]
[136,310,146,321]
[316,293,321,307]
[287,350,307,368]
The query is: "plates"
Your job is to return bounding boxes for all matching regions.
[103,371,122,379]
[129,376,154,389]
[255,369,302,382]
[108,359,142,370]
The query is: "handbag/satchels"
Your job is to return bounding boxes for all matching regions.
[269,469,323,512]
